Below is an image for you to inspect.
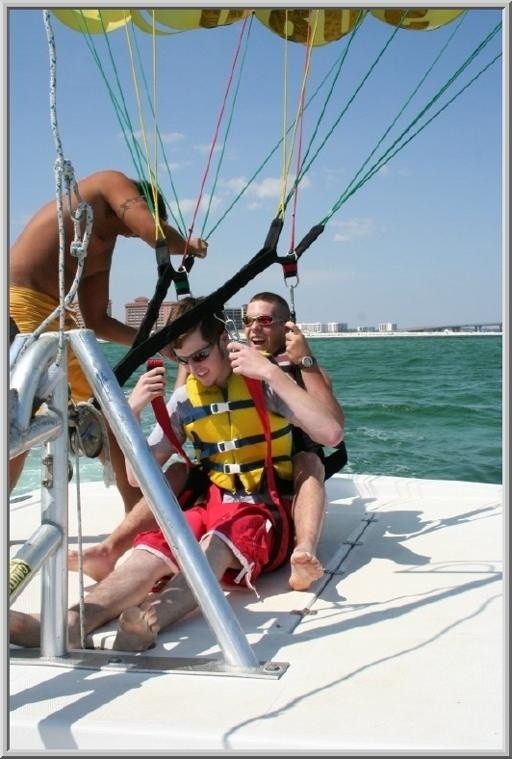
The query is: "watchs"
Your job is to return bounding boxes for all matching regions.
[292,354,319,370]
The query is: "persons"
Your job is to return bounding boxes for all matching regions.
[67,289,346,596]
[8,296,350,653]
[7,166,210,520]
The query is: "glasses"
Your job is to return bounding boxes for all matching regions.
[172,338,216,365]
[241,316,283,326]
